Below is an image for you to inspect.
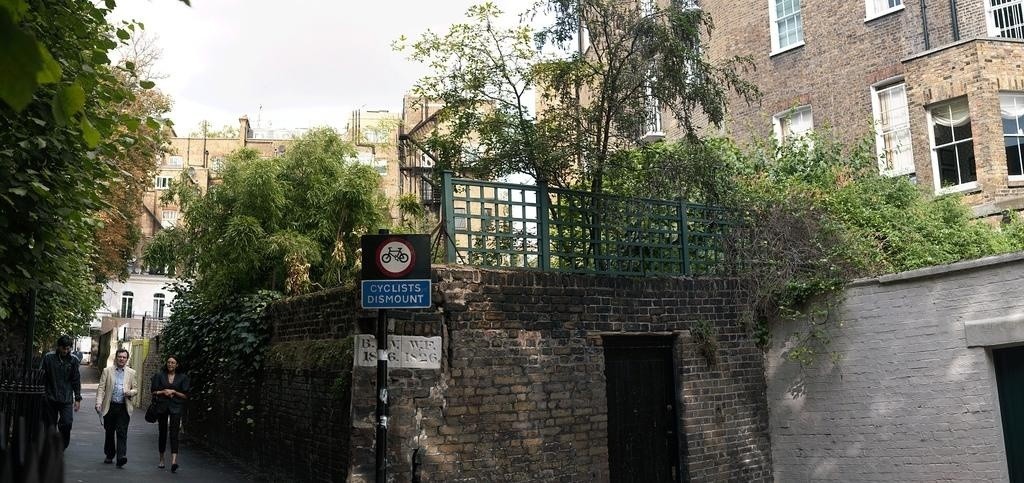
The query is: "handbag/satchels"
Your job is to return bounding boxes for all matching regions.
[145,403,159,423]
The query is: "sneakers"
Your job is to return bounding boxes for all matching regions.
[158,463,165,468]
[171,464,178,472]
[115,456,127,467]
[104,458,112,464]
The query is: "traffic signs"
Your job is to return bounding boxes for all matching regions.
[361,234,432,309]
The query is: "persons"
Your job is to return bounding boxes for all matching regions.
[95,350,139,465]
[41,336,82,452]
[75,347,83,362]
[151,356,189,472]
